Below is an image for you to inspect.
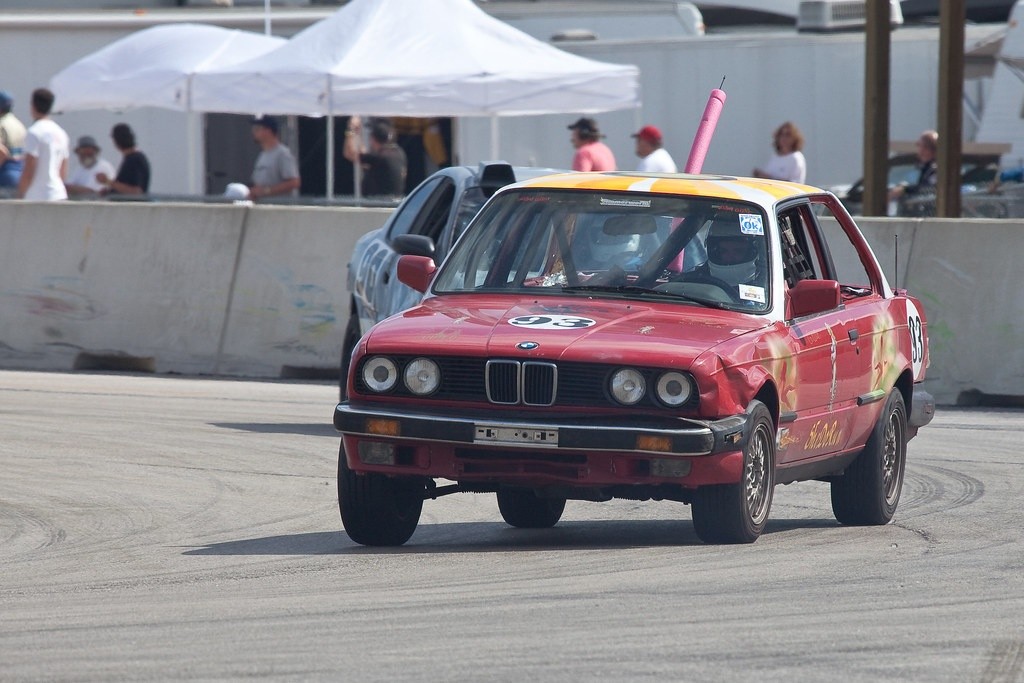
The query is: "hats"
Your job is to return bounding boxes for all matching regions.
[566,118,599,141]
[74,137,99,155]
[372,122,393,143]
[247,113,278,130]
[631,127,662,146]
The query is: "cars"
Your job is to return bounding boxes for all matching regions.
[341,160,710,402]
[336,172,943,549]
[816,150,1024,220]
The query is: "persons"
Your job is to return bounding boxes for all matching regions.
[630,125,677,262]
[754,121,806,232]
[567,118,616,172]
[887,131,940,217]
[245,114,302,206]
[985,99,1024,194]
[342,116,409,201]
[679,212,765,293]
[575,211,652,270]
[0,88,150,202]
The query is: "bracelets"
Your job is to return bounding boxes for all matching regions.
[344,129,356,138]
[262,186,272,196]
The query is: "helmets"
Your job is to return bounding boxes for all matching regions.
[706,221,760,287]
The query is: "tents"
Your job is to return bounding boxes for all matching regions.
[189,0,640,205]
[48,0,290,195]
